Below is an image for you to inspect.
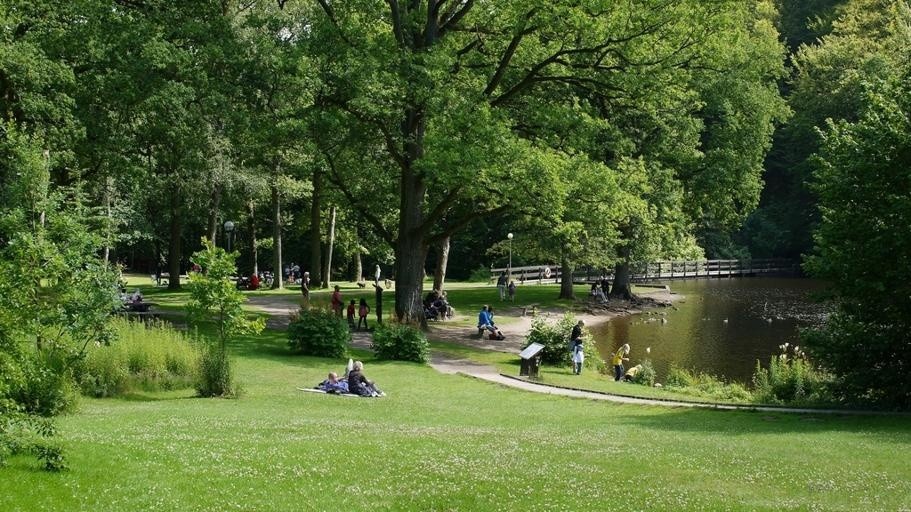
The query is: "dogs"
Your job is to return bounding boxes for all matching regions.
[357,282,366,289]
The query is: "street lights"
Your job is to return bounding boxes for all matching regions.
[507,231,514,285]
[223,220,235,254]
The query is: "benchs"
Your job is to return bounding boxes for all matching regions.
[520,302,541,316]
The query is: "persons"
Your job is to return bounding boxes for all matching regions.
[591,276,610,303]
[302,272,311,305]
[331,285,344,317]
[285,261,301,281]
[237,273,259,290]
[357,298,370,329]
[192,264,203,271]
[326,358,383,395]
[374,264,381,286]
[497,272,516,304]
[423,289,453,322]
[347,300,355,328]
[479,305,506,340]
[624,364,642,382]
[613,344,630,381]
[568,320,585,375]
